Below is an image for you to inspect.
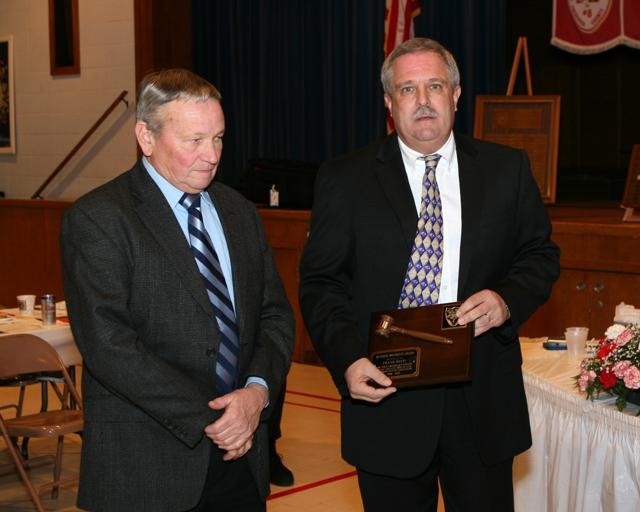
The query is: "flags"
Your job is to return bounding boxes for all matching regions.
[382,0,422,137]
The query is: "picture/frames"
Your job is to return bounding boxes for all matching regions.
[0,34,16,154]
[473,95,560,204]
[48,0,80,75]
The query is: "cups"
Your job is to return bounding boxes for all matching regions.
[17,294,36,317]
[565,326,589,363]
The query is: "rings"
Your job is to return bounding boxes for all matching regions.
[485,314,490,323]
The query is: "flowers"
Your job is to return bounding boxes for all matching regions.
[571,302,640,412]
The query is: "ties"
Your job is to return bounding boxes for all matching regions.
[397,154,444,310]
[178,192,240,394]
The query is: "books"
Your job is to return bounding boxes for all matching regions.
[366,301,474,390]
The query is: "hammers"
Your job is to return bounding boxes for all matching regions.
[374,315,454,344]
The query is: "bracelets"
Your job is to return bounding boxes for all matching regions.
[504,302,512,321]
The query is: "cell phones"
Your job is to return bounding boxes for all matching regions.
[543,339,567,352]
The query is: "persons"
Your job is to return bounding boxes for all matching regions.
[59,67,296,512]
[300,36,560,512]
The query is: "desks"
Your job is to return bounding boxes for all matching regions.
[252,208,640,365]
[512,341,640,512]
[0,199,75,308]
[0,305,82,410]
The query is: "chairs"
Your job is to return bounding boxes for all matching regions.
[0,334,84,512]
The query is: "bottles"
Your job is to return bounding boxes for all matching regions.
[42,294,56,325]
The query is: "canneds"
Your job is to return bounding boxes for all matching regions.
[40,294,54,325]
